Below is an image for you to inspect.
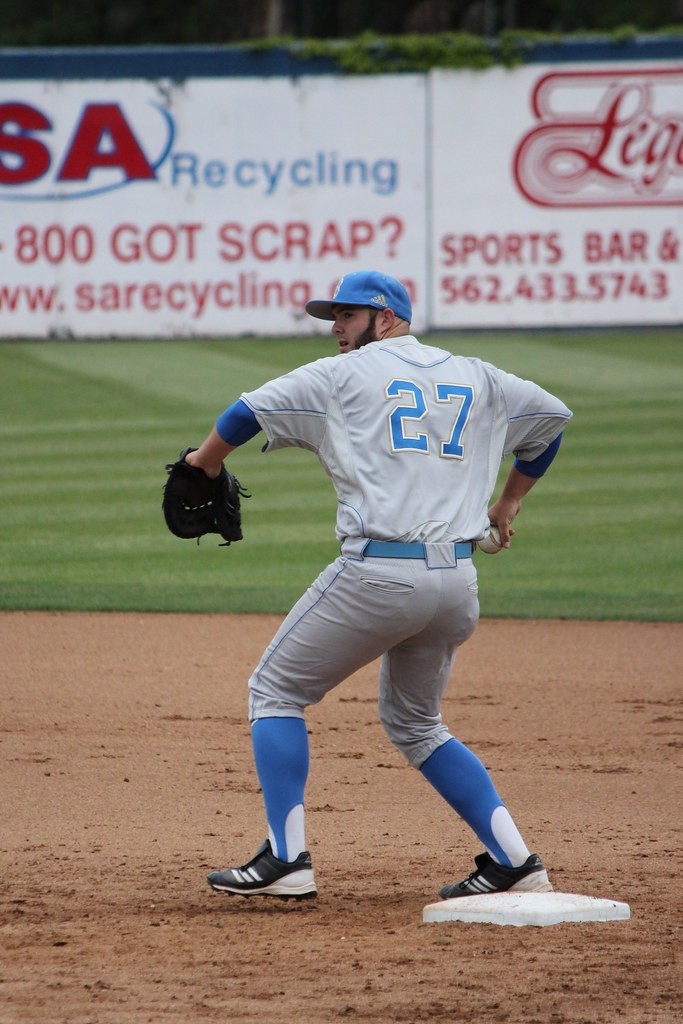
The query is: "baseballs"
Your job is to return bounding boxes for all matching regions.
[476,525,503,553]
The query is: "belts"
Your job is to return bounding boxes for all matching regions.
[363,539,472,559]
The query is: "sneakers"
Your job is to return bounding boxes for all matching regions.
[438,852,554,900]
[206,839,318,898]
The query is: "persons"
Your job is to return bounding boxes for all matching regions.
[161,272,574,898]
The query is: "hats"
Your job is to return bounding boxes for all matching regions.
[304,270,412,325]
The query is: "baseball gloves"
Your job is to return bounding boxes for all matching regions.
[162,448,250,546]
